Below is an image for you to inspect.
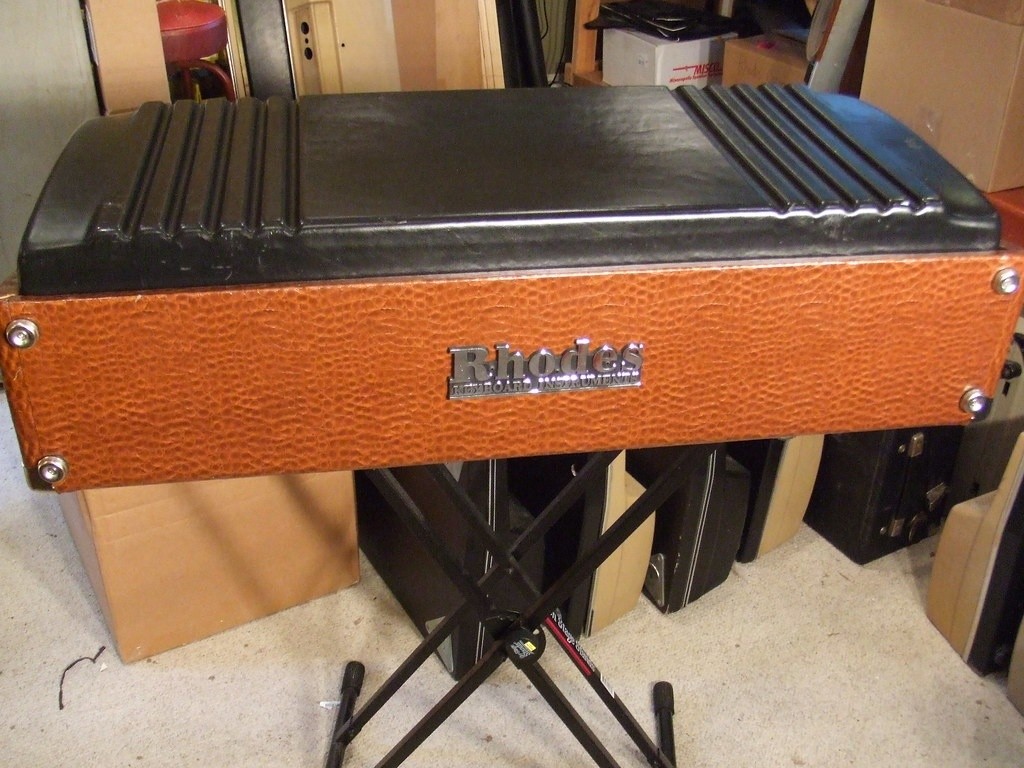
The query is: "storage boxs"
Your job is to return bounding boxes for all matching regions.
[56,472,363,663]
[602,28,737,94]
[722,34,811,87]
[859,0,1024,195]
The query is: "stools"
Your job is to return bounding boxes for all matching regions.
[157,0,237,103]
